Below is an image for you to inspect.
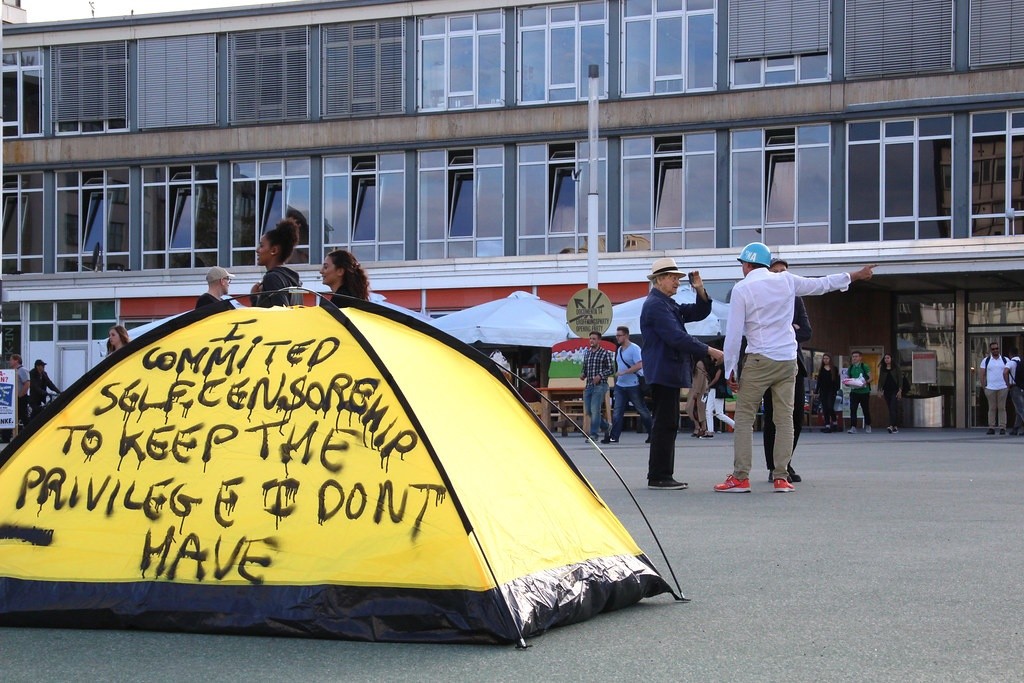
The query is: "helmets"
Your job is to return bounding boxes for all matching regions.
[736,242,771,268]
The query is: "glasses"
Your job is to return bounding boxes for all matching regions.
[991,348,999,351]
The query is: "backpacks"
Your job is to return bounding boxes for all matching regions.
[1008,359,1024,389]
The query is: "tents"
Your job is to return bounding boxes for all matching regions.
[0,287,689,651]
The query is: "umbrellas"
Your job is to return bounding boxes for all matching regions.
[427,287,581,348]
[603,284,731,338]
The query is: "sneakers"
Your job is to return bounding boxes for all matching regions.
[714,474,752,493]
[648,478,686,490]
[585,429,713,444]
[774,479,796,491]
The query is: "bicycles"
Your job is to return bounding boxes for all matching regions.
[18,393,61,426]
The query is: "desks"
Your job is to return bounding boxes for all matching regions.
[534,387,612,437]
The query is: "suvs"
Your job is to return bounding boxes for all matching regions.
[761,377,843,414]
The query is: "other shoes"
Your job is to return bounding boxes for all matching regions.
[1009,430,1018,435]
[768,467,801,482]
[987,429,995,434]
[1019,432,1024,435]
[831,426,840,432]
[893,429,898,434]
[1000,429,1005,435]
[866,426,871,433]
[821,428,831,433]
[847,428,857,433]
[887,427,892,433]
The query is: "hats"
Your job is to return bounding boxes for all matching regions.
[646,258,686,281]
[771,258,789,268]
[35,360,48,367]
[205,266,235,282]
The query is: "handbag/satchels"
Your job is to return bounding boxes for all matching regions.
[715,382,733,398]
[902,376,910,397]
[638,376,649,398]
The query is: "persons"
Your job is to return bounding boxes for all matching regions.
[686,355,735,441]
[979,342,1016,435]
[600,326,654,444]
[878,354,903,433]
[319,250,370,309]
[813,353,841,433]
[902,373,911,395]
[104,324,130,355]
[250,217,304,308]
[196,266,237,310]
[713,242,880,494]
[762,257,813,483]
[639,258,723,489]
[1003,346,1024,435]
[9,355,61,427]
[578,331,614,444]
[845,351,874,434]
